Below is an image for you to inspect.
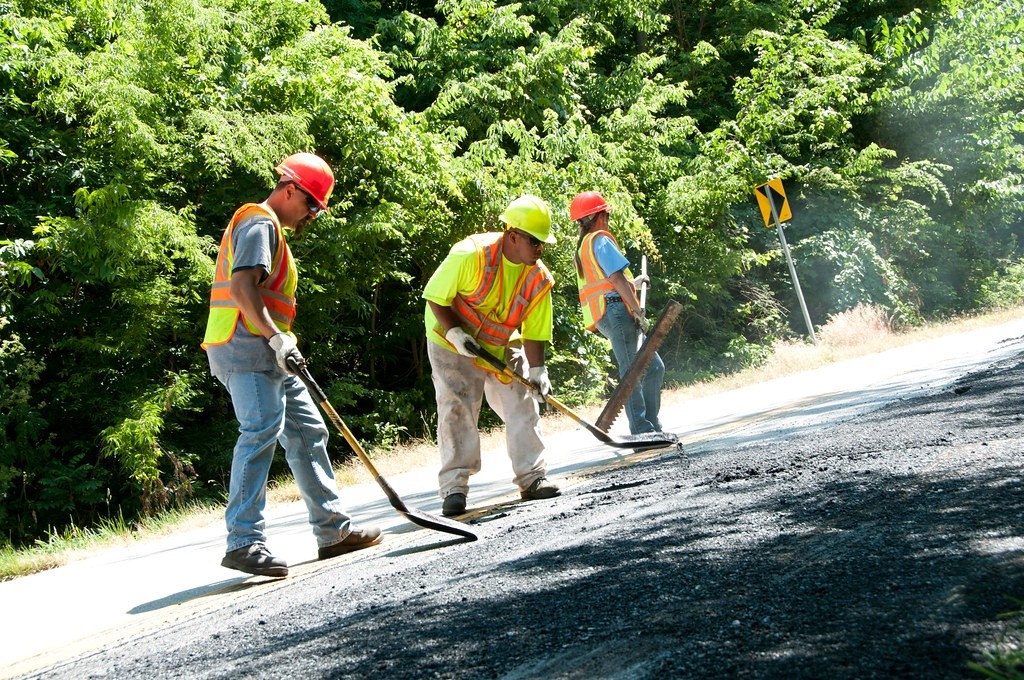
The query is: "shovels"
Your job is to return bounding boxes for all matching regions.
[286,352,480,540]
[463,338,678,450]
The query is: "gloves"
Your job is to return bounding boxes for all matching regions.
[268,331,304,378]
[445,327,480,358]
[632,310,650,336]
[634,274,650,290]
[528,366,553,402]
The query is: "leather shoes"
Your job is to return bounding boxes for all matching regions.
[318,527,384,560]
[222,543,288,577]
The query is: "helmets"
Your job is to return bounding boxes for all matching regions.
[570,191,608,220]
[274,152,335,211]
[499,195,557,244]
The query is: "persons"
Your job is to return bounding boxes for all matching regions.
[421,197,562,514]
[200,153,384,577]
[570,191,679,450]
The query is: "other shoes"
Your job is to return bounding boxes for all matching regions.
[521,476,561,500]
[633,431,678,453]
[442,492,466,515]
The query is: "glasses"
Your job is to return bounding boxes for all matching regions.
[295,186,321,213]
[509,231,545,247]
[603,212,609,220]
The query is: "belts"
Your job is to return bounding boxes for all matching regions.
[606,296,623,304]
